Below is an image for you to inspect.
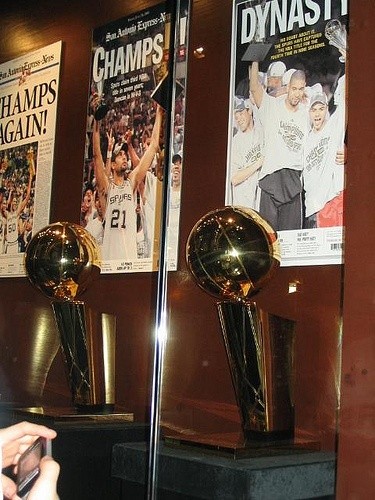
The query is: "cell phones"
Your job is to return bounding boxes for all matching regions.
[17,436,51,497]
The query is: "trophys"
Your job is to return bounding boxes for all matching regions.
[92,62,113,118]
[8,221,135,423]
[189,208,290,450]
[324,17,346,52]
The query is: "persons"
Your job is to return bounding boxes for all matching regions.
[0,142,41,255]
[228,45,346,233]
[1,419,61,500]
[82,77,184,267]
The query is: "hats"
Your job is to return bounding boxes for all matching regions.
[82,184,94,202]
[111,142,128,162]
[281,68,297,86]
[172,150,182,164]
[308,92,328,110]
[257,71,267,90]
[234,99,251,113]
[266,61,287,79]
